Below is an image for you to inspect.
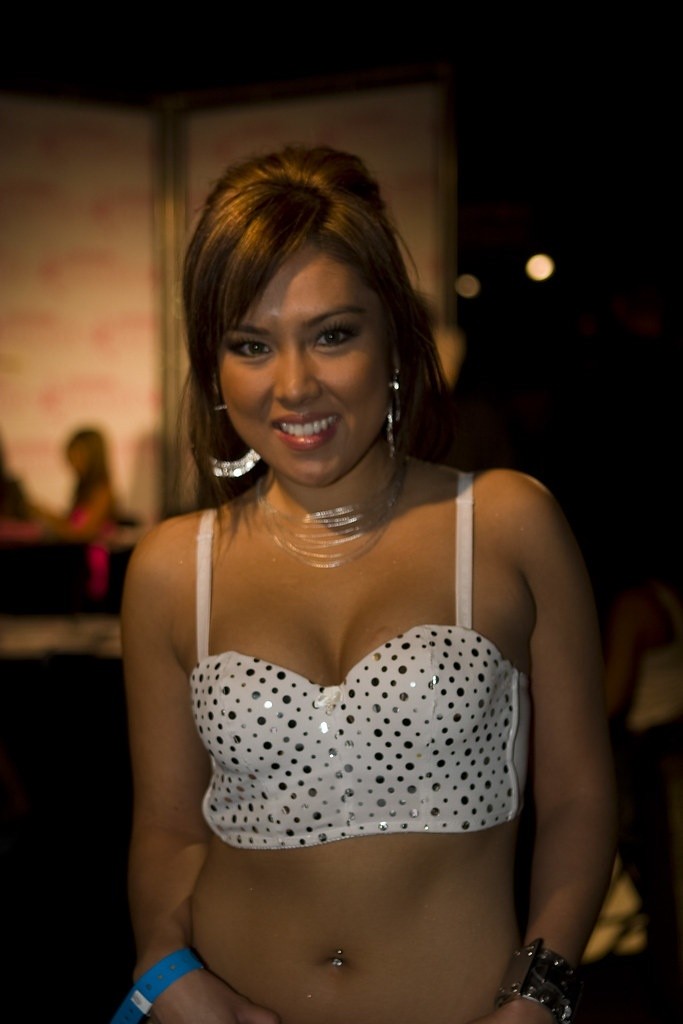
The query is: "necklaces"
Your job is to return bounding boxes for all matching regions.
[254,442,404,570]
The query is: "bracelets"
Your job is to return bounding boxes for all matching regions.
[497,939,583,1024]
[111,947,205,1024]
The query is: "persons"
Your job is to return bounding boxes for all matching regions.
[0,442,53,521]
[126,144,620,1024]
[602,514,683,913]
[59,427,143,593]
